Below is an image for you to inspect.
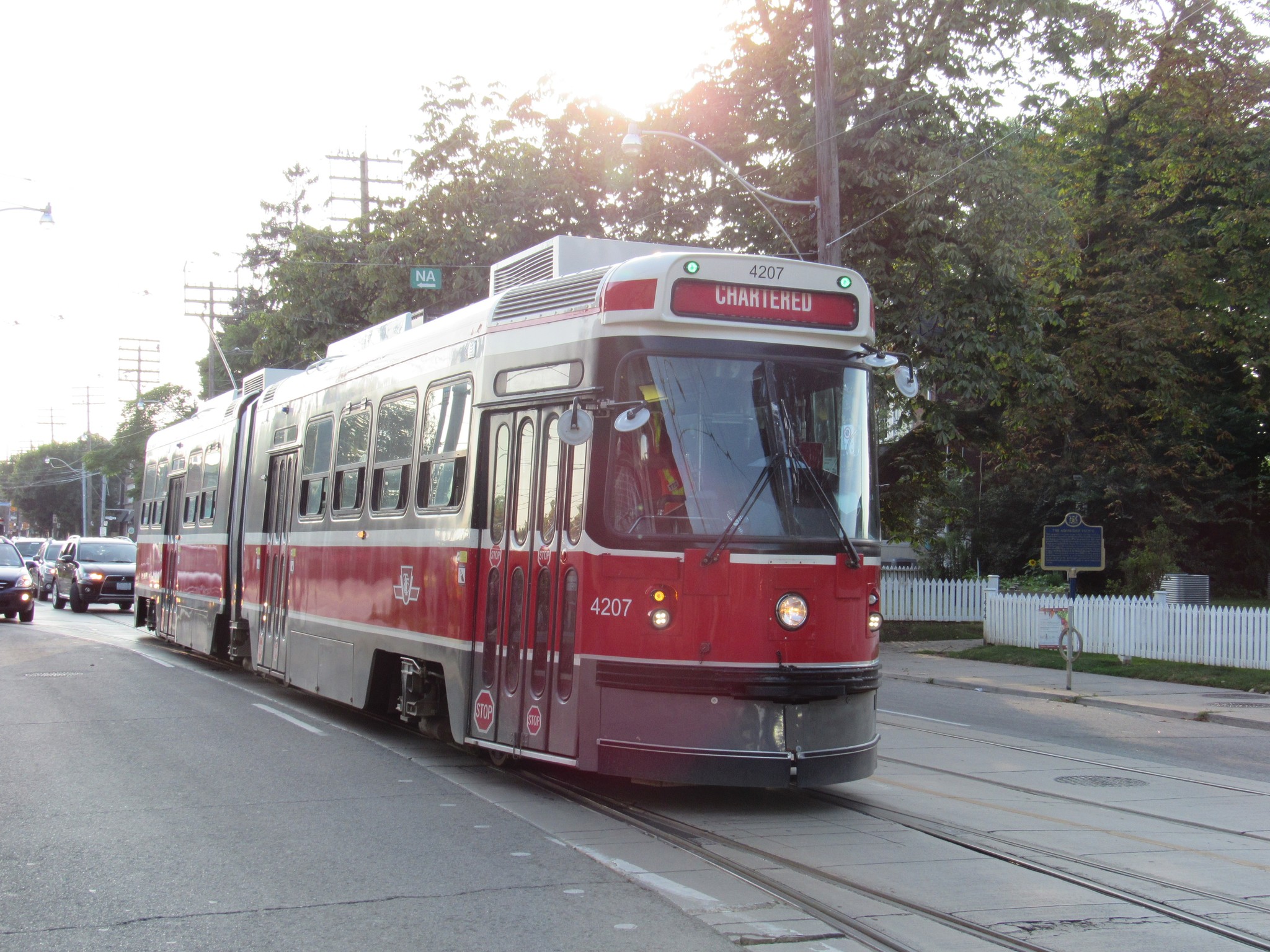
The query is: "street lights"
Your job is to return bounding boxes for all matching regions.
[622,131,841,265]
[46,457,88,537]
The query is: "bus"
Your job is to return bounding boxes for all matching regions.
[132,234,920,791]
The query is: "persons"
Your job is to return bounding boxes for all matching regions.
[651,441,698,533]
[748,421,811,469]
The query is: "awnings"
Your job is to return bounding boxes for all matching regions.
[124,510,134,523]
[114,510,128,522]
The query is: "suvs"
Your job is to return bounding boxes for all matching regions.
[0,536,38,622]
[31,537,73,602]
[10,536,49,572]
[53,535,138,614]
